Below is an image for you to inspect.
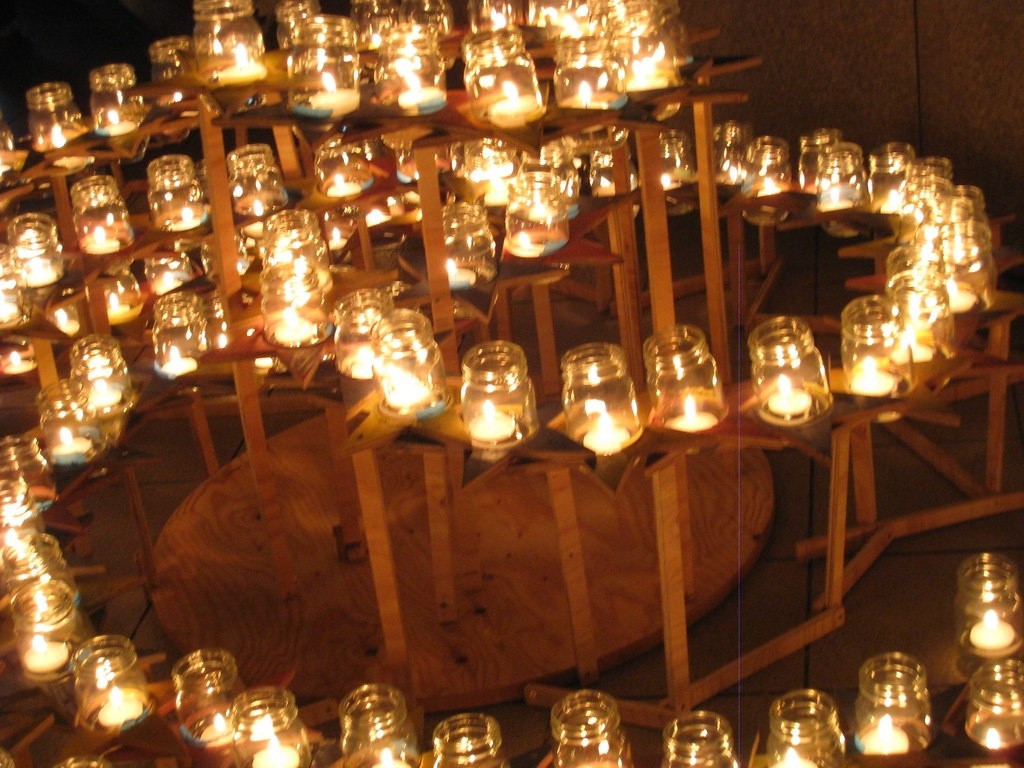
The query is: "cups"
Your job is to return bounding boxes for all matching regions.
[0,0,1024,768]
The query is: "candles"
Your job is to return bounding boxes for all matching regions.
[0,0,1024,768]
